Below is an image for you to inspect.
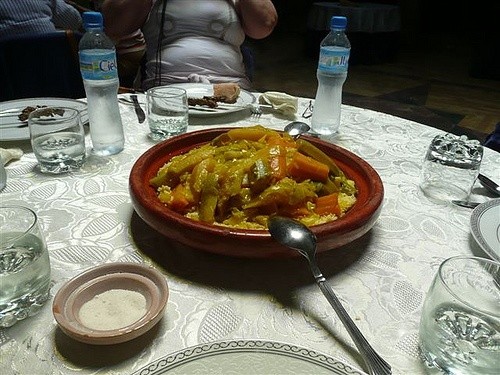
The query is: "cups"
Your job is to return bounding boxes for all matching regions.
[419,133,483,202]
[147,86,188,141]
[28,106,86,174]
[0,205,52,329]
[417,255,500,375]
[0,153,7,193]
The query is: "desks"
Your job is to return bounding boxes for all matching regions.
[0,93,499,375]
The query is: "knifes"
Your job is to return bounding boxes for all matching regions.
[452,200,481,208]
[130,95,146,123]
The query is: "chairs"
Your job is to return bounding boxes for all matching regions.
[0,30,86,100]
[97,0,159,92]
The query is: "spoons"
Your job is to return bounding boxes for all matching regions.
[267,211,392,375]
[284,122,320,140]
[477,173,500,197]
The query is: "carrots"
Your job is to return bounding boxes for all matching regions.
[266,134,286,180]
[290,194,340,217]
[170,182,189,210]
[287,153,330,183]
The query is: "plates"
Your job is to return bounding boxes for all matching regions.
[128,128,384,258]
[0,97,90,141]
[52,261,168,345]
[469,199,500,263]
[148,82,256,117]
[128,338,369,375]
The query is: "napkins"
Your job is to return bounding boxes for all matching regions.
[259,92,298,114]
[0,147,23,165]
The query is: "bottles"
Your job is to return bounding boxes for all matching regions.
[78,12,125,156]
[311,16,351,136]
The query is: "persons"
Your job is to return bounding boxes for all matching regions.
[0,0,83,33]
[102,0,278,90]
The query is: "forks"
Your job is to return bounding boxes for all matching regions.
[249,106,263,115]
